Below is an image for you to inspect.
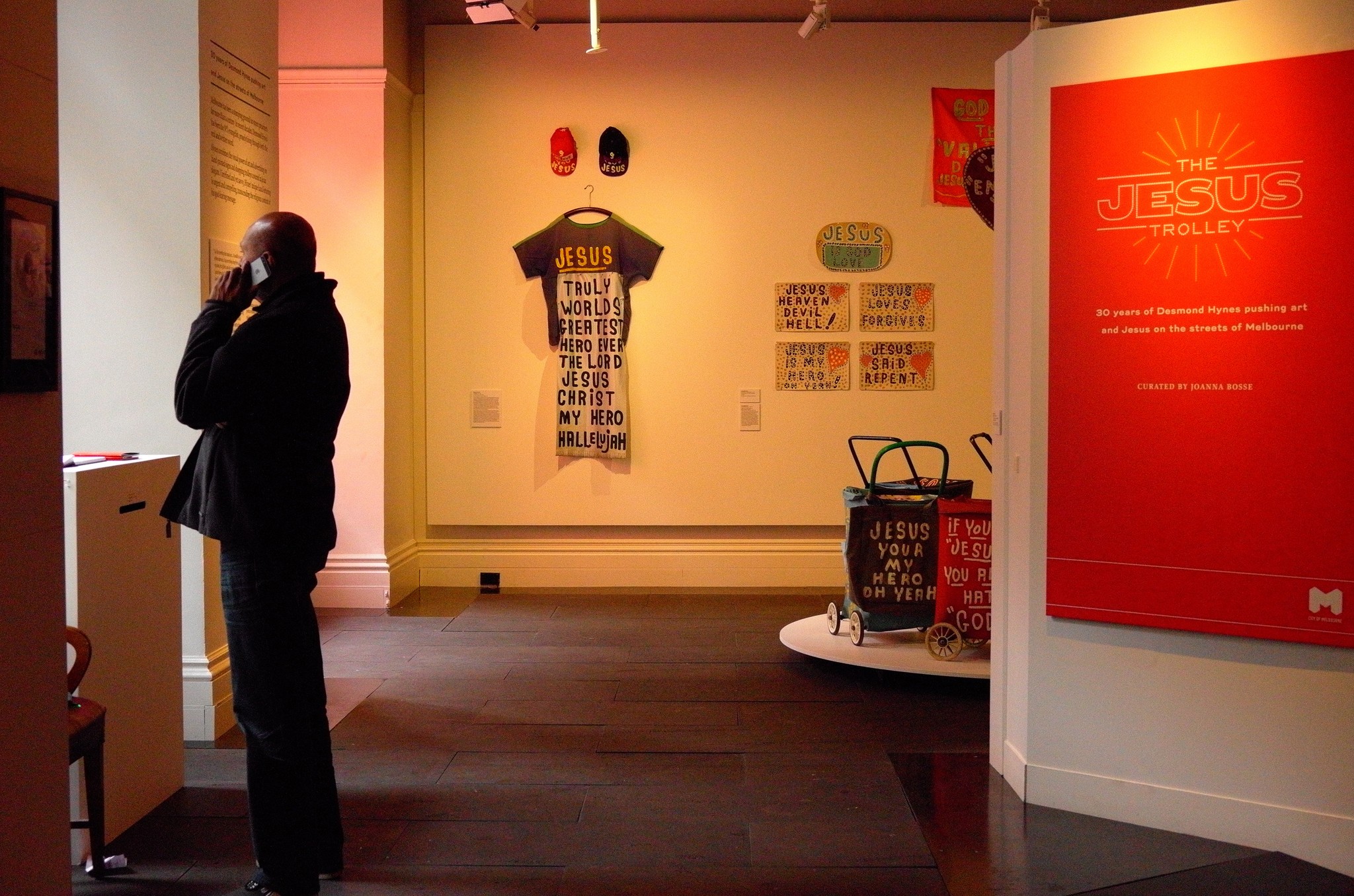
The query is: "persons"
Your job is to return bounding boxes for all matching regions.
[155,212,348,893]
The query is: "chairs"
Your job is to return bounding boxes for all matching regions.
[67,625,106,883]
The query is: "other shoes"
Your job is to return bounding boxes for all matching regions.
[221,881,281,896]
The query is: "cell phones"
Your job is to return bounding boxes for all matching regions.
[240,256,272,290]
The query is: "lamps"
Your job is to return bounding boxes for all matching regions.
[511,0,540,32]
[1029,1,1049,33]
[797,0,833,39]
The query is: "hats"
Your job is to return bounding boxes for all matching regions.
[550,127,577,177]
[599,125,630,177]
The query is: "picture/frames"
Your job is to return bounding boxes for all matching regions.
[0,187,62,396]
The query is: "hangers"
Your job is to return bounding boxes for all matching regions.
[562,185,611,220]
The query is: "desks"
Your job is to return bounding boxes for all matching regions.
[61,449,181,862]
